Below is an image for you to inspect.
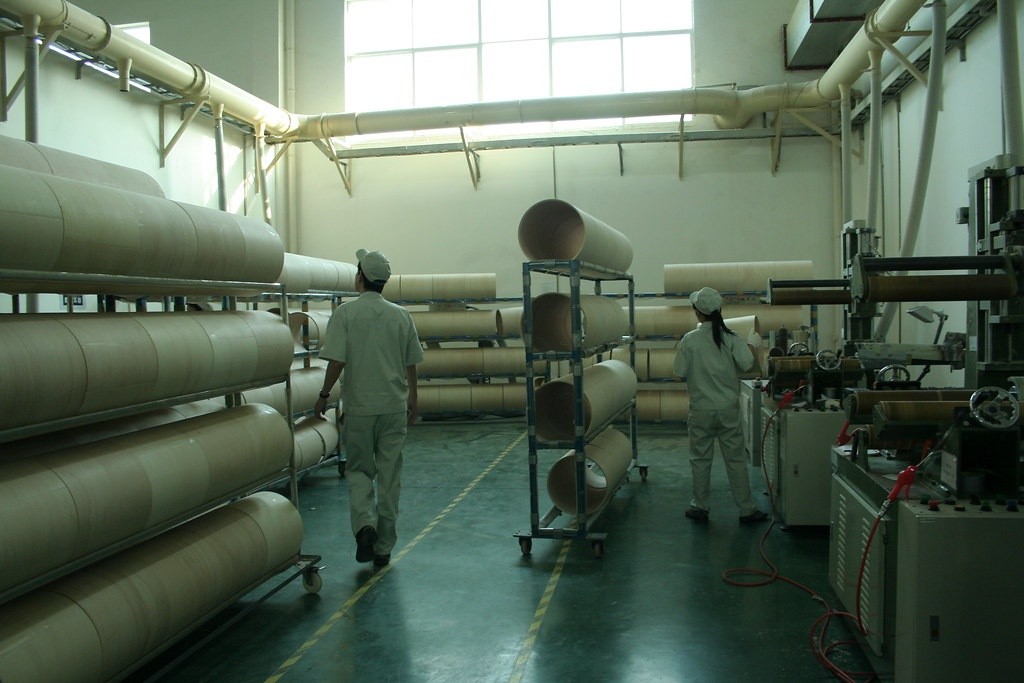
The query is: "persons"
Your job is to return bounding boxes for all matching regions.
[314,248,424,568]
[672,287,769,524]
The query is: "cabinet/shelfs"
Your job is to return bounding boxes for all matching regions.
[389,299,527,417]
[602,288,819,423]
[513,258,649,557]
[0,272,359,683]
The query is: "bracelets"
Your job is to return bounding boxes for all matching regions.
[319,391,330,398]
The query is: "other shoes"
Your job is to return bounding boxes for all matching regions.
[373,554,391,566]
[356,526,376,563]
[686,507,708,521]
[739,510,768,524]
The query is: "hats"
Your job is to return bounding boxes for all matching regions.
[356,249,391,285]
[690,287,722,315]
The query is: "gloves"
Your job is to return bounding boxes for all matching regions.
[747,328,762,350]
